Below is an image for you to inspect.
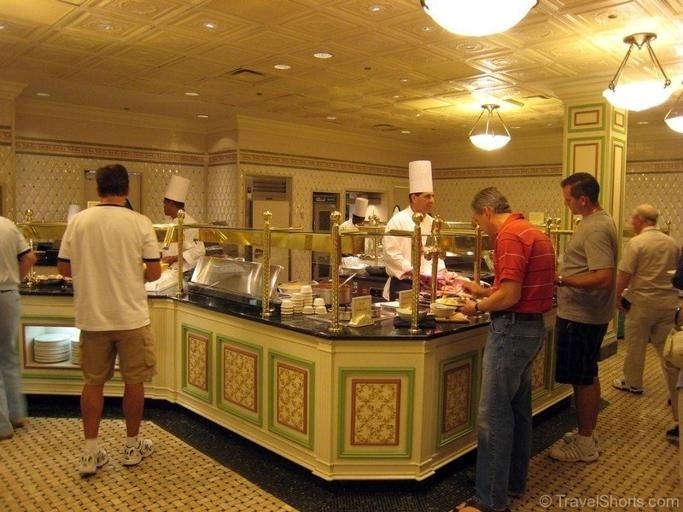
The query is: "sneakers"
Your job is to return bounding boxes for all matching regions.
[563,431,600,446]
[613,378,644,395]
[124,438,154,466]
[79,449,110,474]
[548,441,600,464]
[665,424,680,442]
[11,417,29,430]
[0,429,13,440]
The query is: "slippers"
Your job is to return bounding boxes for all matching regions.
[448,498,513,512]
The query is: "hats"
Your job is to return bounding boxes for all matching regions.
[353,196,370,218]
[408,159,434,194]
[163,174,190,203]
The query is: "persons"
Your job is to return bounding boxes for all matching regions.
[548,172,618,463]
[663,247,683,442]
[163,174,206,276]
[457,187,556,512]
[340,198,369,228]
[55,164,162,476]
[0,216,37,439]
[613,204,683,394]
[382,160,457,299]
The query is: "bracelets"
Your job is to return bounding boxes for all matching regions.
[558,274,563,286]
[475,303,478,312]
[673,307,680,325]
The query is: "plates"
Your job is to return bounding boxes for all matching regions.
[435,297,480,307]
[33,334,79,363]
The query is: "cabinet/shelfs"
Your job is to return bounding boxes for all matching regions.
[18,315,122,397]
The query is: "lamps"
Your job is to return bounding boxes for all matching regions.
[602,32,676,111]
[421,0,538,37]
[664,81,683,133]
[468,105,512,149]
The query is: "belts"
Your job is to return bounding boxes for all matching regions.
[491,311,541,322]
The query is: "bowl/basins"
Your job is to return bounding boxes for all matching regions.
[337,307,351,321]
[33,241,59,264]
[317,272,357,305]
[396,307,425,322]
[429,303,454,318]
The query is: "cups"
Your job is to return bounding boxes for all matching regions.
[370,306,381,317]
[279,284,326,317]
[34,274,60,284]
